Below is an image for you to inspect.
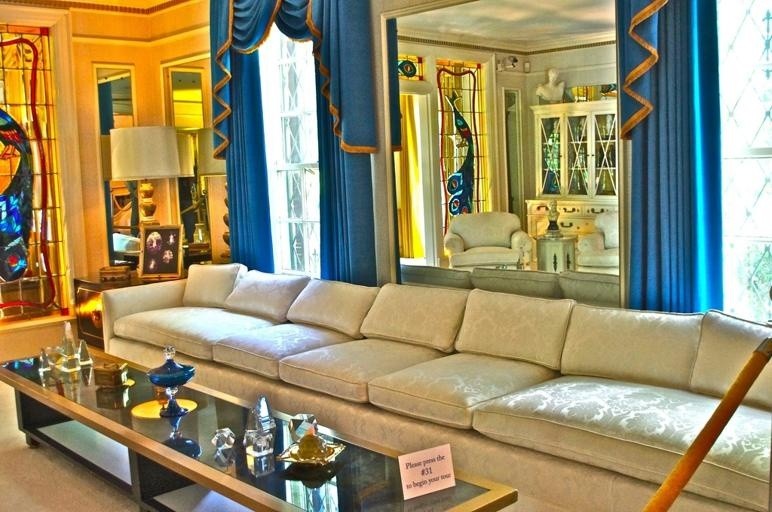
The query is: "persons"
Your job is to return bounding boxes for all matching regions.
[535,67,566,103]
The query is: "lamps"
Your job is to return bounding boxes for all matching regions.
[198,129,230,257]
[496,56,518,72]
[175,133,195,177]
[110,126,181,275]
[100,135,112,182]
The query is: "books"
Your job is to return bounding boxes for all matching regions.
[573,84,600,103]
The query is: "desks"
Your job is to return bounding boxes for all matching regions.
[0,341,518,512]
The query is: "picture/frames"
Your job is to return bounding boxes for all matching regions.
[138,224,183,282]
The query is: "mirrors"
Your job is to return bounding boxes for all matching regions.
[92,63,140,267]
[160,51,230,269]
[168,67,206,245]
[381,0,628,308]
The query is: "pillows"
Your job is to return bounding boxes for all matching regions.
[225,270,309,320]
[360,282,469,354]
[690,309,772,408]
[186,264,242,306]
[288,278,380,342]
[455,288,574,372]
[560,300,704,392]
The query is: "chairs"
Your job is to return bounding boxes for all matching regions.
[577,212,619,276]
[444,211,532,272]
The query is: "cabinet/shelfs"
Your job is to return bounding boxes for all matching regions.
[524,99,619,263]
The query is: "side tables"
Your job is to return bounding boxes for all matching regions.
[74,277,187,349]
[533,235,577,274]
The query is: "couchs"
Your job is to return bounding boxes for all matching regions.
[101,280,772,512]
